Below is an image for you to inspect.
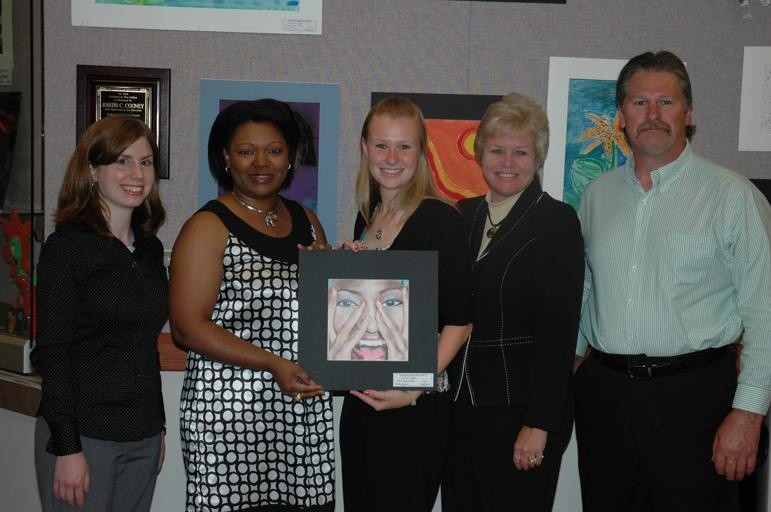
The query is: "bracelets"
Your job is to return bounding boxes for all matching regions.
[405,391,417,406]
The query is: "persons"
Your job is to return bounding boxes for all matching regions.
[339,96,473,512]
[31,113,169,512]
[327,279,409,362]
[441,93,585,512]
[573,50,771,512]
[169,98,336,512]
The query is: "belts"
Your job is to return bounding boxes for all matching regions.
[589,358,730,383]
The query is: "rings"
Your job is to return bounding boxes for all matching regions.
[513,456,521,460]
[293,393,305,403]
[529,457,537,464]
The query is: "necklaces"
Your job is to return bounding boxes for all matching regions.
[487,205,509,239]
[375,230,383,241]
[230,191,282,229]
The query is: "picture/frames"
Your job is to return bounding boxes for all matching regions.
[71,0,323,35]
[371,91,505,206]
[542,56,687,208]
[76,65,172,179]
[297,249,439,390]
[199,77,340,247]
